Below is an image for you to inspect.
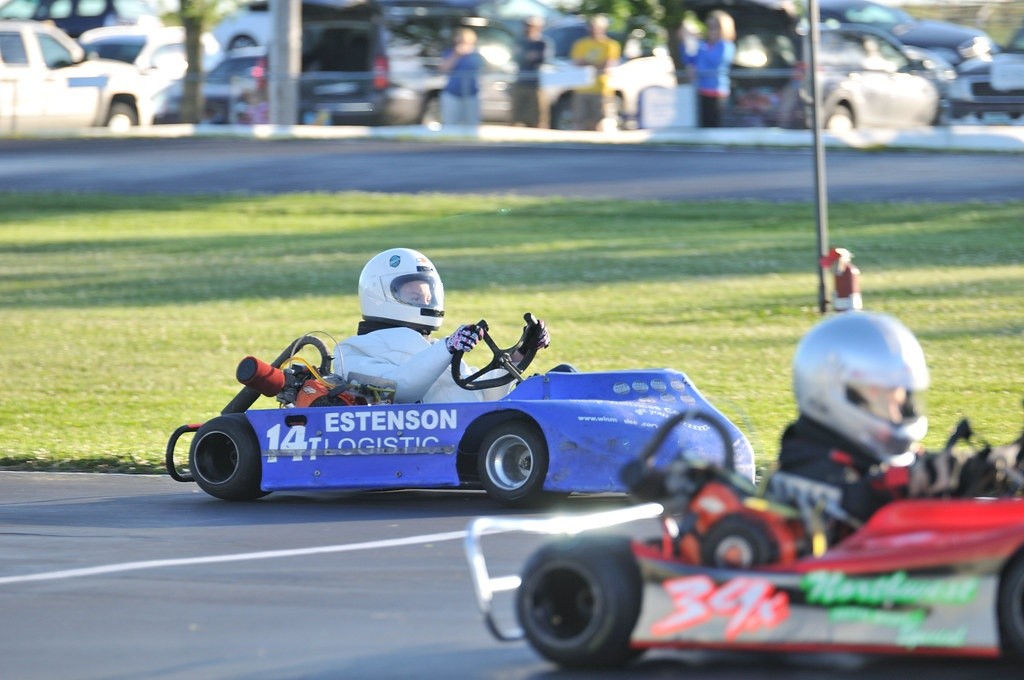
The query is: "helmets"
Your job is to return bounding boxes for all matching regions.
[791,309,930,467]
[357,248,445,336]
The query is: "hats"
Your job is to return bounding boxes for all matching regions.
[522,15,544,27]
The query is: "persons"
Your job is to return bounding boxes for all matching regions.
[332,248,550,404]
[780,310,1024,561]
[571,13,621,130]
[678,11,736,127]
[511,15,556,128]
[438,26,482,126]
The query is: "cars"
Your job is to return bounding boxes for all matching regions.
[0,0,1024,130]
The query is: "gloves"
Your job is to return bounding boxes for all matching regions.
[446,324,485,352]
[517,319,551,356]
[907,448,976,498]
